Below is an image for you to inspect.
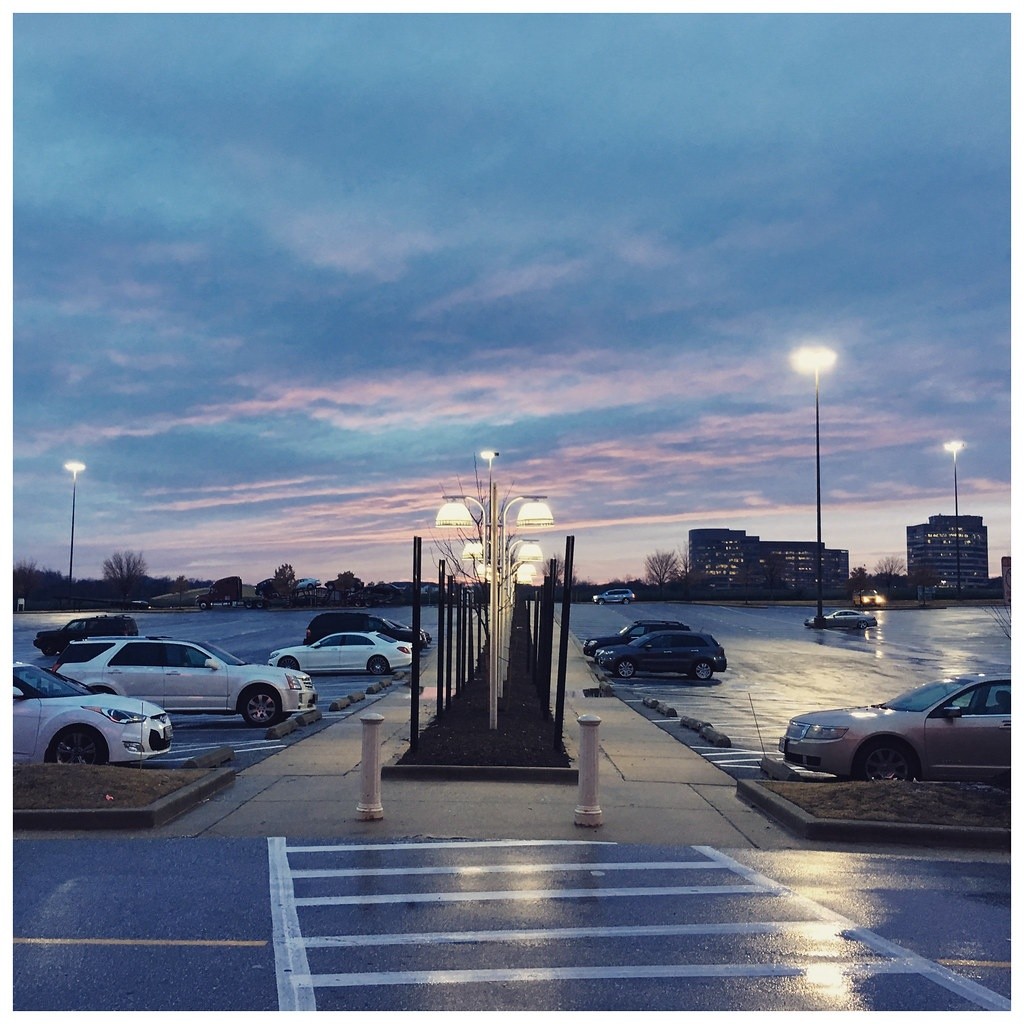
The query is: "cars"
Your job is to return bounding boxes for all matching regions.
[195,575,438,610]
[778,673,1011,788]
[804,609,877,630]
[853,590,880,607]
[385,617,432,643]
[13,661,176,768]
[266,630,413,676]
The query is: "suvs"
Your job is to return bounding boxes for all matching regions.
[592,588,635,606]
[302,611,427,655]
[583,619,692,658]
[33,615,139,657]
[52,637,318,727]
[596,630,727,681]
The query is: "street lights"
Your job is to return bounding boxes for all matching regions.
[947,440,963,601]
[63,459,86,611]
[799,347,833,627]
[434,449,555,732]
